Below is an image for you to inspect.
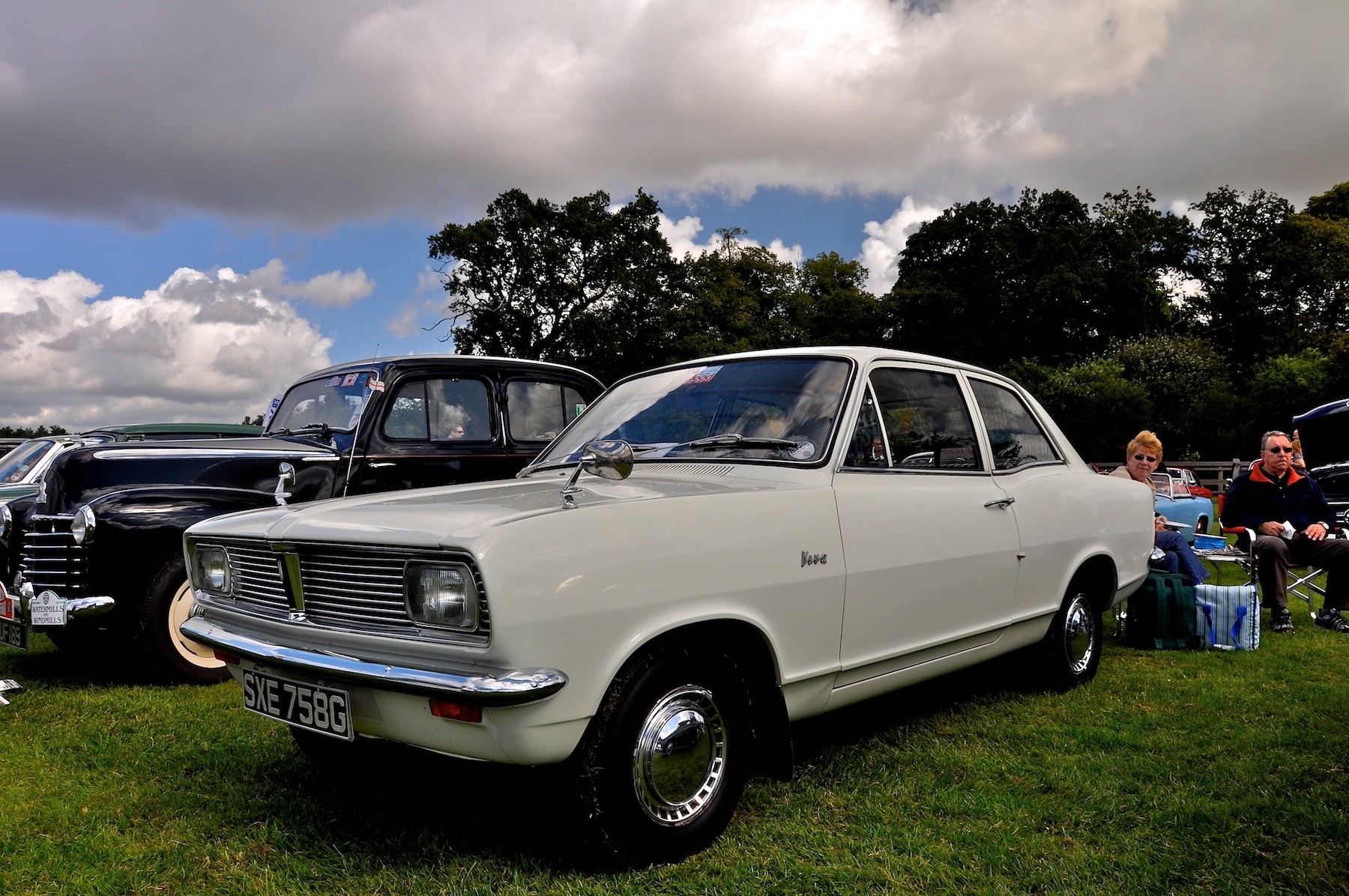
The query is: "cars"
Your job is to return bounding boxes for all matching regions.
[1292,399,1349,529]
[0,422,267,580]
[177,343,1156,867]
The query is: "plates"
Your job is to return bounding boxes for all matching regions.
[1157,520,1192,528]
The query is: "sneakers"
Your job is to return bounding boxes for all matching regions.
[1315,607,1349,634]
[1271,612,1294,632]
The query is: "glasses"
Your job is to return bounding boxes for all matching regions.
[1130,453,1158,463]
[1262,447,1293,453]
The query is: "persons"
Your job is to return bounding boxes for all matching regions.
[1289,428,1308,475]
[1222,429,1349,634]
[1107,430,1211,588]
[446,424,464,439]
[865,437,887,467]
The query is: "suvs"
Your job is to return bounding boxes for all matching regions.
[0,353,630,685]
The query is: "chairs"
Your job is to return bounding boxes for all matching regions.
[1216,494,1346,629]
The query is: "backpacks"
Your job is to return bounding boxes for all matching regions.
[1124,575,1198,651]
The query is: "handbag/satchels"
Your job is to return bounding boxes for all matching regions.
[1193,583,1260,651]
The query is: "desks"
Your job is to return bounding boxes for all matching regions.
[1190,546,1252,587]
